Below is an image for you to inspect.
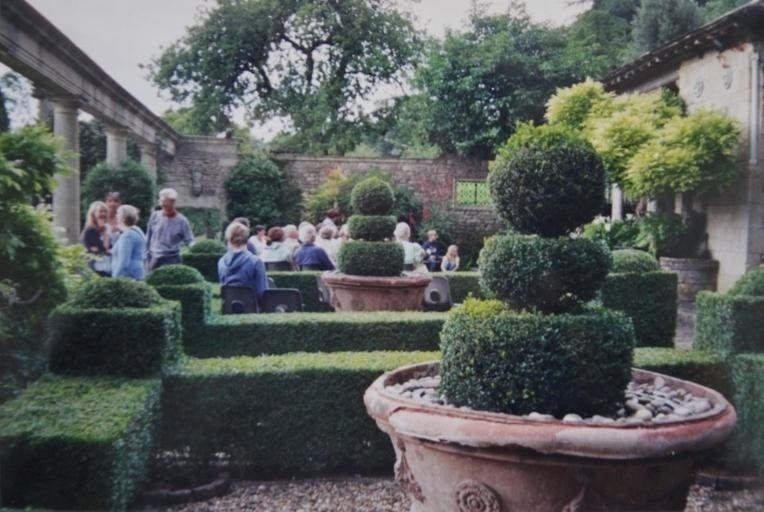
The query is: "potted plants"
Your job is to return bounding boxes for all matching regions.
[318,174,429,311]
[363,127,739,509]
[629,115,744,306]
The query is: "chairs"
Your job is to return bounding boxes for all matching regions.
[299,263,323,270]
[401,255,443,271]
[220,275,451,315]
[264,261,292,270]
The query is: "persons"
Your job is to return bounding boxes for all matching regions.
[218,222,283,312]
[145,188,196,271]
[78,201,113,277]
[100,191,122,246]
[110,204,146,281]
[234,208,461,272]
[151,205,162,213]
